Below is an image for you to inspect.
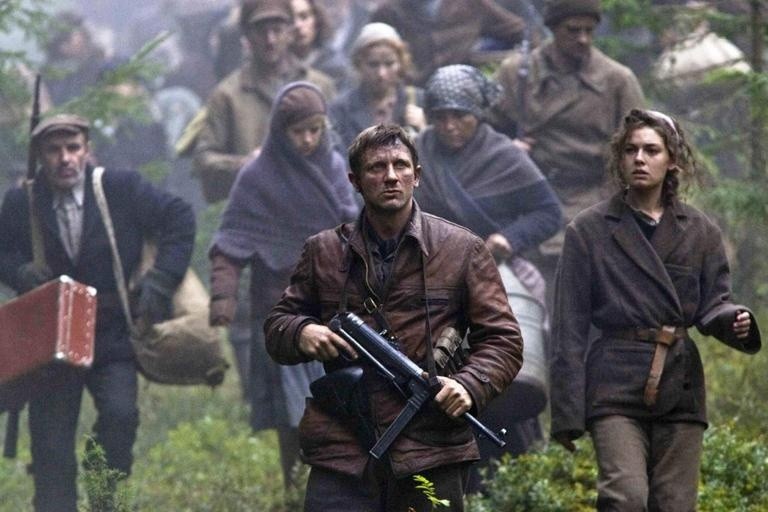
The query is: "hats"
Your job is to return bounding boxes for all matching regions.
[29,112,91,141]
[238,2,296,28]
[543,0,603,26]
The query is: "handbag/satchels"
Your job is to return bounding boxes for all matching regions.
[125,240,230,387]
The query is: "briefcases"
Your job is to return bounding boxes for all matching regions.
[2,274,97,383]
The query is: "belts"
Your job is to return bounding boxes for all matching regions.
[605,322,694,408]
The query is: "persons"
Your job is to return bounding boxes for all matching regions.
[263,126,522,512]
[0,112,196,511]
[204,83,358,494]
[175,4,654,308]
[550,107,762,510]
[402,69,567,464]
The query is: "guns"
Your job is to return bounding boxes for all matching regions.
[3,72,42,458]
[324,311,506,459]
